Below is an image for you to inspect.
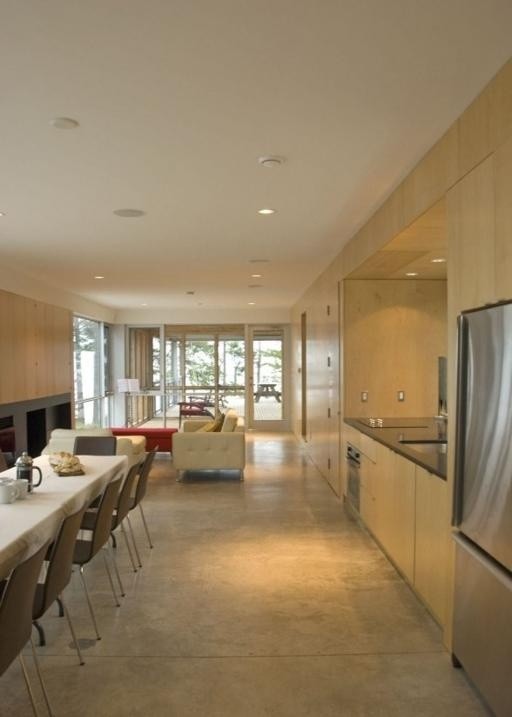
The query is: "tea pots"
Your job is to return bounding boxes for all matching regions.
[15,452,42,492]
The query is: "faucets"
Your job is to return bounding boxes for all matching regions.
[433,415,448,422]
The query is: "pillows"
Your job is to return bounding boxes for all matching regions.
[197,407,225,432]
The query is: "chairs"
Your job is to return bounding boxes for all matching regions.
[188,383,229,409]
[1,501,90,715]
[77,460,141,599]
[40,471,120,642]
[81,450,156,566]
[72,436,117,454]
[0,535,56,714]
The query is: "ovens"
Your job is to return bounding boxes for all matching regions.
[340,442,365,515]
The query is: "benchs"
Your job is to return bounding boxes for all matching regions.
[111,428,178,455]
[254,392,281,403]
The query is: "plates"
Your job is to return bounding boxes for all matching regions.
[48,457,85,477]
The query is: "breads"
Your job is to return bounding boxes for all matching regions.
[48,451,83,474]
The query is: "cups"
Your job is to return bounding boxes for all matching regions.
[0,476,32,504]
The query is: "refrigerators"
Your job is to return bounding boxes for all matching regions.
[451,299,512,717]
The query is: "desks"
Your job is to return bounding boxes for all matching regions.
[1,450,128,578]
[255,384,281,403]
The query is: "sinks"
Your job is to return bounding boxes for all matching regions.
[398,439,448,456]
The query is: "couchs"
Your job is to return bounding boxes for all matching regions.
[171,406,246,482]
[40,428,146,470]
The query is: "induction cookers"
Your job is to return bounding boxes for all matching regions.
[356,415,427,429]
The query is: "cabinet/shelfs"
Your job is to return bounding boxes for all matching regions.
[361,431,447,629]
